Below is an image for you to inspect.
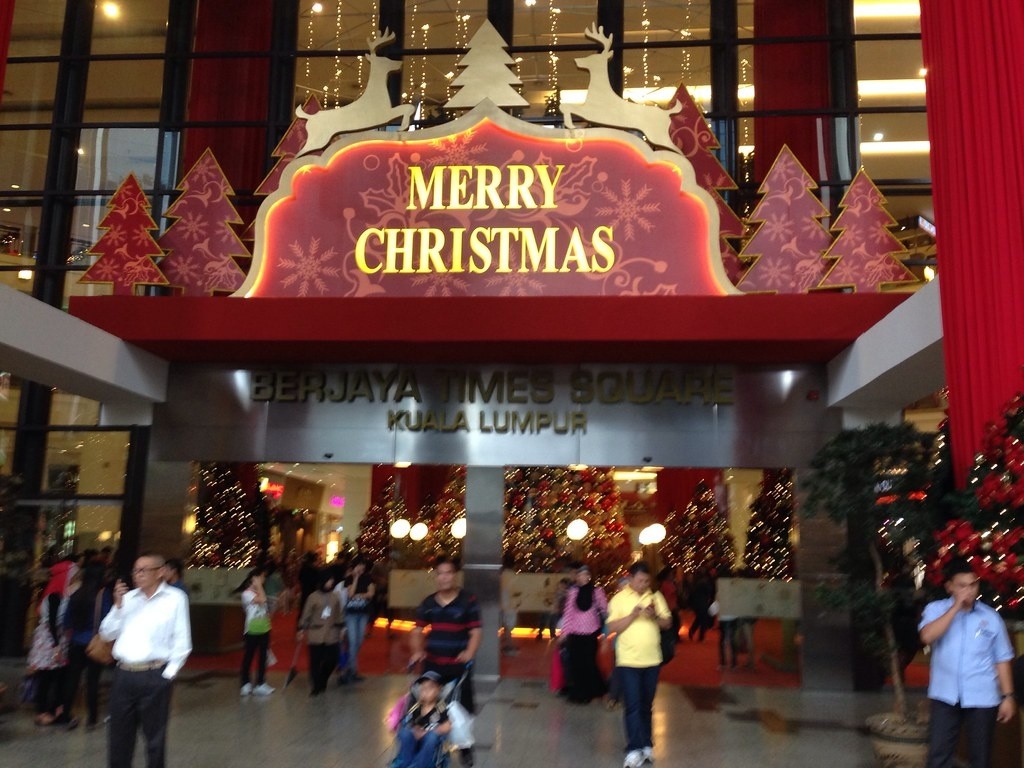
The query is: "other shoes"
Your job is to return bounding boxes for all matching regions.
[310,685,327,697]
[336,670,366,685]
[716,664,736,672]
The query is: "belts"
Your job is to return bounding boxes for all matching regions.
[115,660,164,672]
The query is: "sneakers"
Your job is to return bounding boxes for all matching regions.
[623,747,654,768]
[240,683,252,696]
[254,683,276,695]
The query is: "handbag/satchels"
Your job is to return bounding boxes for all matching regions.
[86,633,115,665]
[266,650,277,667]
[347,594,367,610]
[446,701,475,750]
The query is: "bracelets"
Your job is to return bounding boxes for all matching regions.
[1001,691,1015,699]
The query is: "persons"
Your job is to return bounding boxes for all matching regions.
[387,555,484,768]
[604,560,673,767]
[235,567,277,698]
[97,552,194,768]
[296,550,377,698]
[917,562,1016,768]
[28,544,189,734]
[498,550,608,706]
[656,556,759,672]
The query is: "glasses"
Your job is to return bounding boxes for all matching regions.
[132,566,160,573]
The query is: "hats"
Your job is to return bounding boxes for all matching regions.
[414,671,440,683]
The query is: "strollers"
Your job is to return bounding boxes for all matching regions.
[386,658,477,768]
